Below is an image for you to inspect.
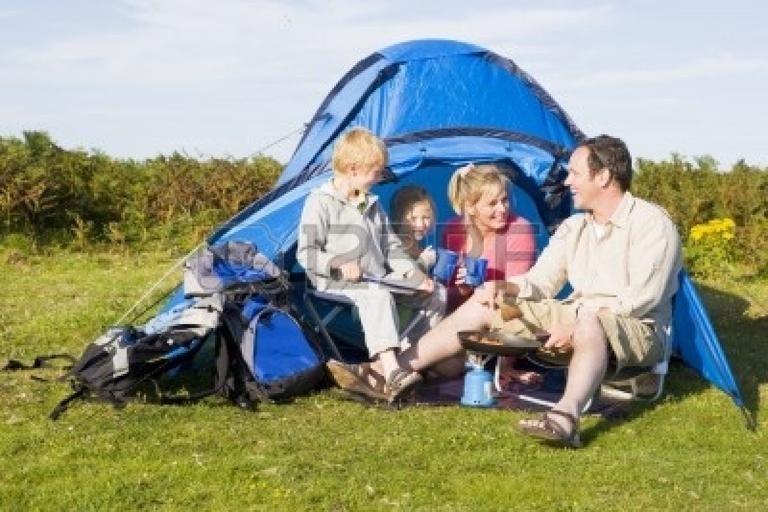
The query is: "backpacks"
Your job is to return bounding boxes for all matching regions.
[70,292,233,404]
[184,238,334,411]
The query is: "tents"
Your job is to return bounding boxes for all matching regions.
[114,38,754,431]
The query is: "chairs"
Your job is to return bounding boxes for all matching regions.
[301,273,429,363]
[493,295,675,416]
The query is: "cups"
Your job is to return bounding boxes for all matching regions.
[429,247,459,285]
[460,256,488,287]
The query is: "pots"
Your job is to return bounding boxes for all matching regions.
[455,327,550,358]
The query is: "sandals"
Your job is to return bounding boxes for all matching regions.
[326,359,424,406]
[518,410,584,448]
